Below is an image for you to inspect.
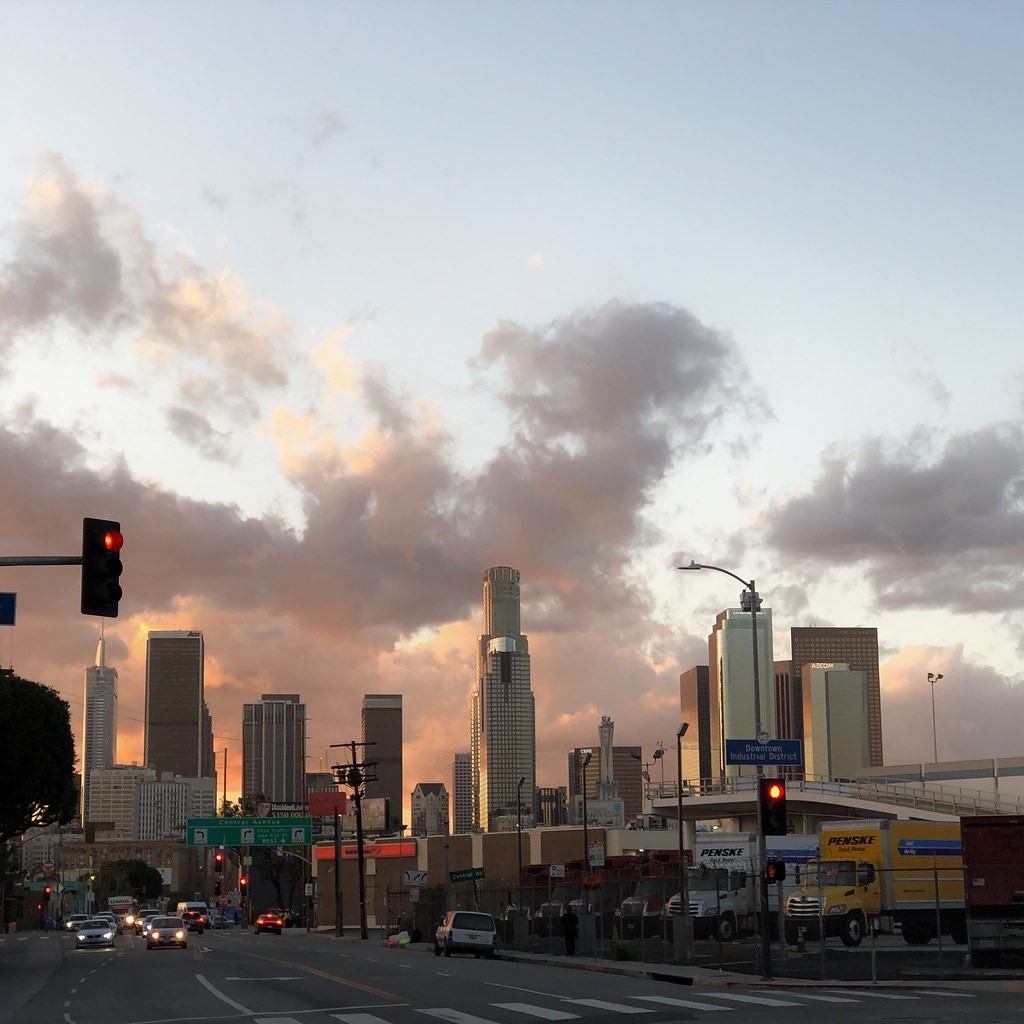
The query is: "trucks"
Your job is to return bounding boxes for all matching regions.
[500,851,693,940]
[658,832,819,945]
[778,814,968,946]
[108,895,135,929]
[177,902,210,929]
[959,813,1024,966]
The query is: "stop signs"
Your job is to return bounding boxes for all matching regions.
[44,859,54,877]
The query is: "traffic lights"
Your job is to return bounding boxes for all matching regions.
[241,877,247,896]
[757,777,788,835]
[37,903,43,911]
[216,902,220,907]
[44,886,50,901]
[764,859,779,883]
[216,851,223,872]
[82,516,122,617]
[240,900,246,908]
[91,873,97,884]
[213,881,221,896]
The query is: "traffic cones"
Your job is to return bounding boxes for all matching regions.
[794,925,809,952]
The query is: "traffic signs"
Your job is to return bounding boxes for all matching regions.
[186,816,313,847]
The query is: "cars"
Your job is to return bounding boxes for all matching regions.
[255,913,282,935]
[58,910,234,949]
[147,917,190,950]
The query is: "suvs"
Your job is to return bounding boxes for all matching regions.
[433,910,497,959]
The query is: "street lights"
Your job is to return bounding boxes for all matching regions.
[516,775,526,915]
[44,841,64,933]
[581,753,593,872]
[927,671,944,763]
[675,722,689,887]
[678,560,774,982]
